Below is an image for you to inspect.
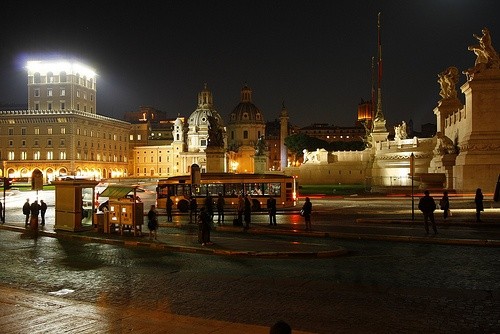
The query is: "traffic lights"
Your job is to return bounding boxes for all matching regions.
[3,178,13,191]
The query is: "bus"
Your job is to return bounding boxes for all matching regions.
[156,172,297,212]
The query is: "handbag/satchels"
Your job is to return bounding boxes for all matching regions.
[233,219,242,227]
[299,210,304,216]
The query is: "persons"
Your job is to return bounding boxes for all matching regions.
[188,195,199,224]
[237,194,244,225]
[22,198,31,226]
[269,320,292,334]
[303,148,321,163]
[440,190,449,222]
[418,190,438,233]
[244,193,253,234]
[29,200,41,230]
[203,192,215,222]
[476,188,484,222]
[217,192,225,223]
[267,194,277,226]
[166,195,173,221]
[199,205,214,247]
[40,200,47,226]
[302,195,312,229]
[394,26,497,154]
[147,204,159,240]
